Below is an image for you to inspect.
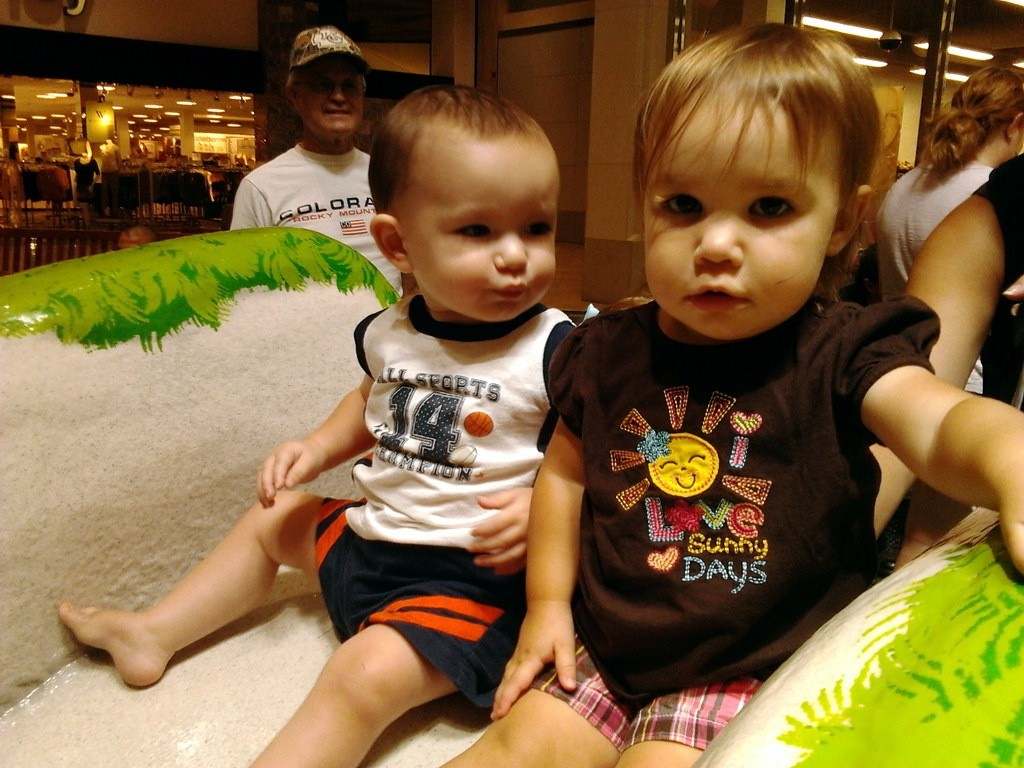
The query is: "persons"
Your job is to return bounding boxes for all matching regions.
[870,156,1023,536]
[876,69,1024,397]
[118,226,157,249]
[67,132,121,225]
[874,86,904,179]
[230,27,420,299]
[442,22,1023,768]
[57,84,575,768]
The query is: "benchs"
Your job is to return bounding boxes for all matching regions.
[0,225,1023,768]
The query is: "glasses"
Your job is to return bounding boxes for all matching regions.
[291,78,367,97]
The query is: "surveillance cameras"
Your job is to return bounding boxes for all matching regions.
[879,30,902,51]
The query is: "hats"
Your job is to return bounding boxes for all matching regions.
[290,25,370,71]
